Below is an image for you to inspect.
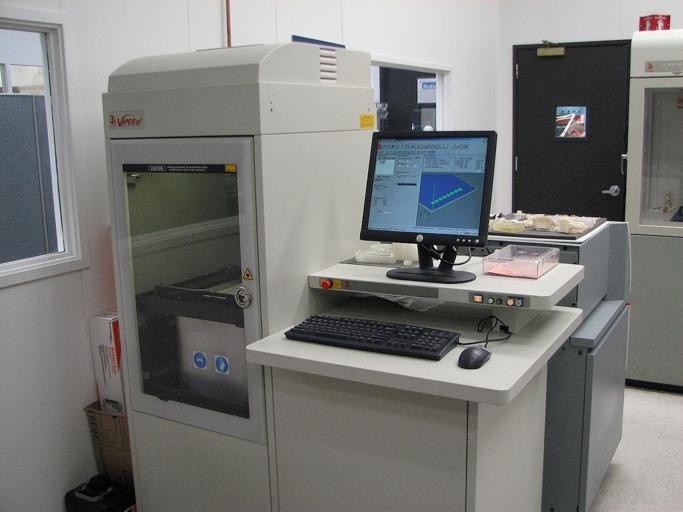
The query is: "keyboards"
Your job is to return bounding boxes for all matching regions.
[284,314,461,362]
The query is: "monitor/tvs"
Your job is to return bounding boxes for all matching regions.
[359,131,498,284]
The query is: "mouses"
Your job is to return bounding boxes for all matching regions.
[458,346,492,369]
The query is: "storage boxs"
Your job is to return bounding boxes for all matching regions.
[81,400,130,491]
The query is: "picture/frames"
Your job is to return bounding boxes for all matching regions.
[552,100,590,142]
